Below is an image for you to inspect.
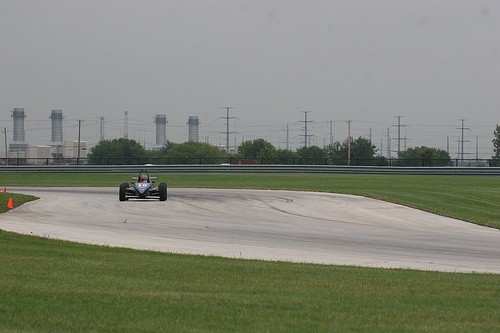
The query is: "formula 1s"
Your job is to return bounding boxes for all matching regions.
[119,170,168,201]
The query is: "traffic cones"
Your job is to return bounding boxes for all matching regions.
[7,197,14,208]
[2,186,7,193]
